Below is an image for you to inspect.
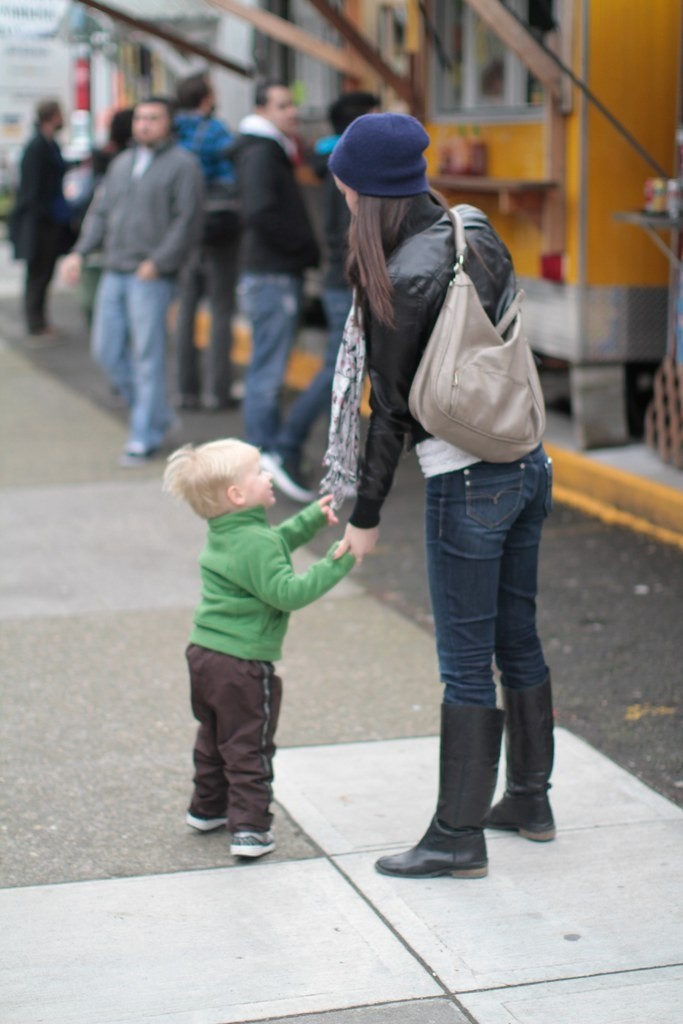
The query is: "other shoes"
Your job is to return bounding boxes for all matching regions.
[262,448,318,502]
[127,437,156,457]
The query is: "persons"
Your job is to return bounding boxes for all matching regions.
[164,438,355,856]
[11,101,92,334]
[321,113,555,878]
[63,73,381,503]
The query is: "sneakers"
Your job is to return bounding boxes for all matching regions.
[186,811,230,831]
[231,831,276,857]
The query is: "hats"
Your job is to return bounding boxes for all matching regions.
[326,112,434,197]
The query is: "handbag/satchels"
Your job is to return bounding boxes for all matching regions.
[407,208,549,462]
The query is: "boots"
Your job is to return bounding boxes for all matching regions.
[375,702,508,880]
[481,666,556,841]
